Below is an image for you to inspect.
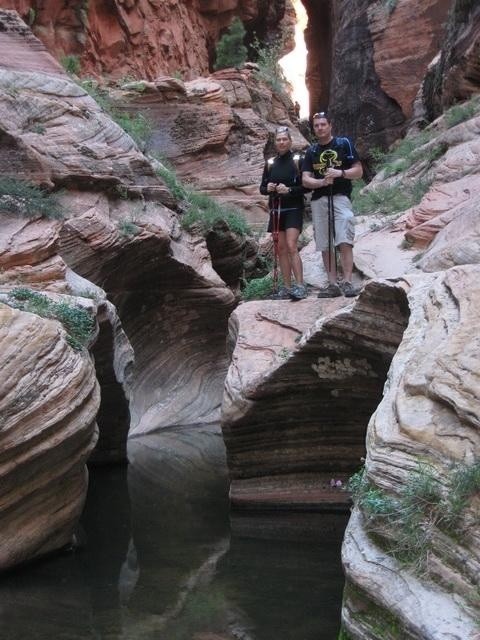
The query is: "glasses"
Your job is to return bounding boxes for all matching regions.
[313,112,325,118]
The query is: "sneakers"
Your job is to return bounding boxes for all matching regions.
[270,287,307,299]
[318,282,355,298]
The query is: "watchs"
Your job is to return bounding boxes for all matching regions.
[341,170,346,179]
[288,187,292,194]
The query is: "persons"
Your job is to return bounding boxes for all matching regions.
[302,112,363,298]
[260,127,312,300]
